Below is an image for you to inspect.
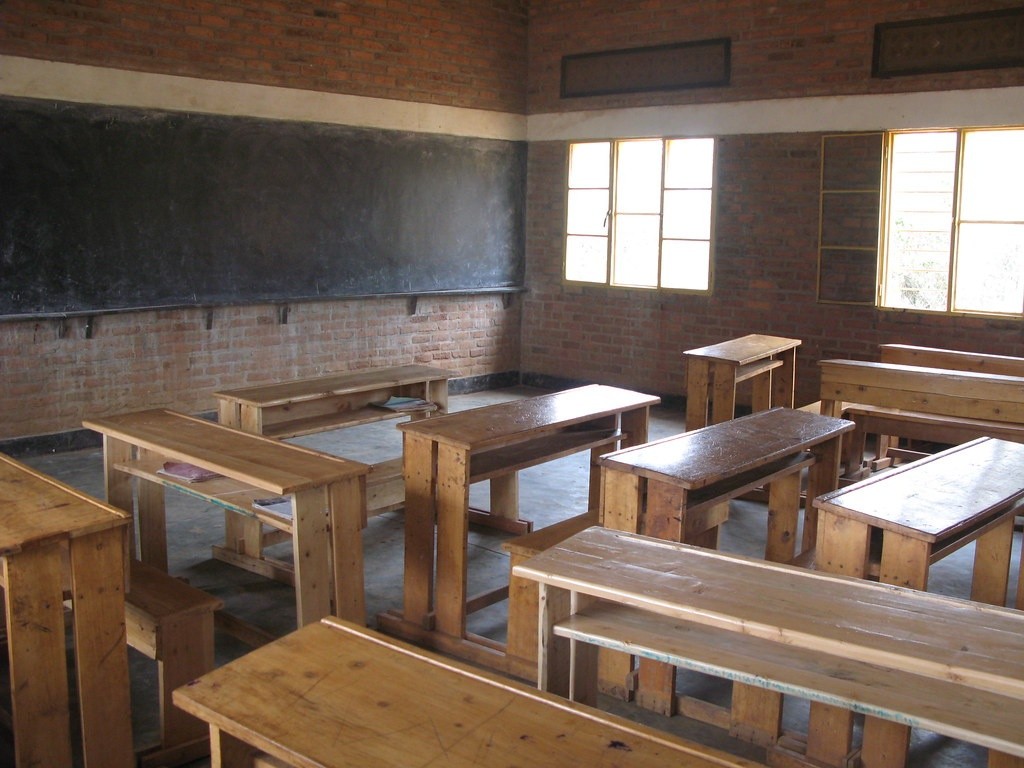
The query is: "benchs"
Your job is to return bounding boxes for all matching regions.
[500,499,730,697]
[795,343,1024,555]
[63,558,228,768]
[362,456,407,516]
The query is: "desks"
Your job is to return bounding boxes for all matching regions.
[594,405,858,719]
[209,365,460,582]
[682,334,802,432]
[514,524,1024,768]
[0,455,134,768]
[376,383,665,661]
[83,408,375,632]
[769,436,1024,768]
[171,614,769,768]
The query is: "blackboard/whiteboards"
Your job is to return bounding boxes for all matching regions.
[0,93,530,321]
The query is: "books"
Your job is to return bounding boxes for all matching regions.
[251,495,329,522]
[368,394,439,414]
[155,461,223,485]
